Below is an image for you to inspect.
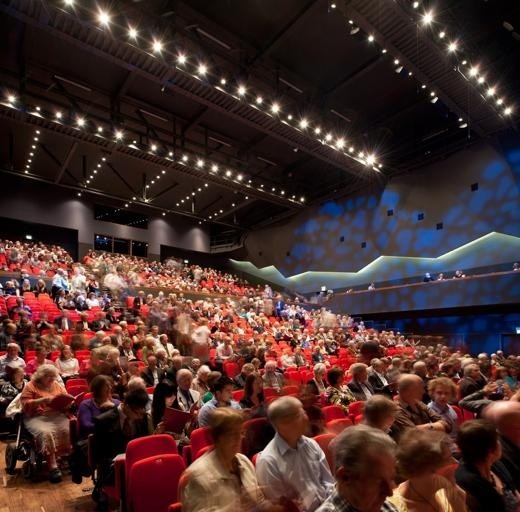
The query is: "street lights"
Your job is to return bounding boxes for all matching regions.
[5,392,24,420]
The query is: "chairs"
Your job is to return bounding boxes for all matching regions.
[1,290,415,507]
[47,466,62,482]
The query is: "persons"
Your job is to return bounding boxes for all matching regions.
[183,408,274,512]
[256,396,334,512]
[389,429,466,512]
[456,419,520,512]
[1,235,520,484]
[318,426,398,512]
